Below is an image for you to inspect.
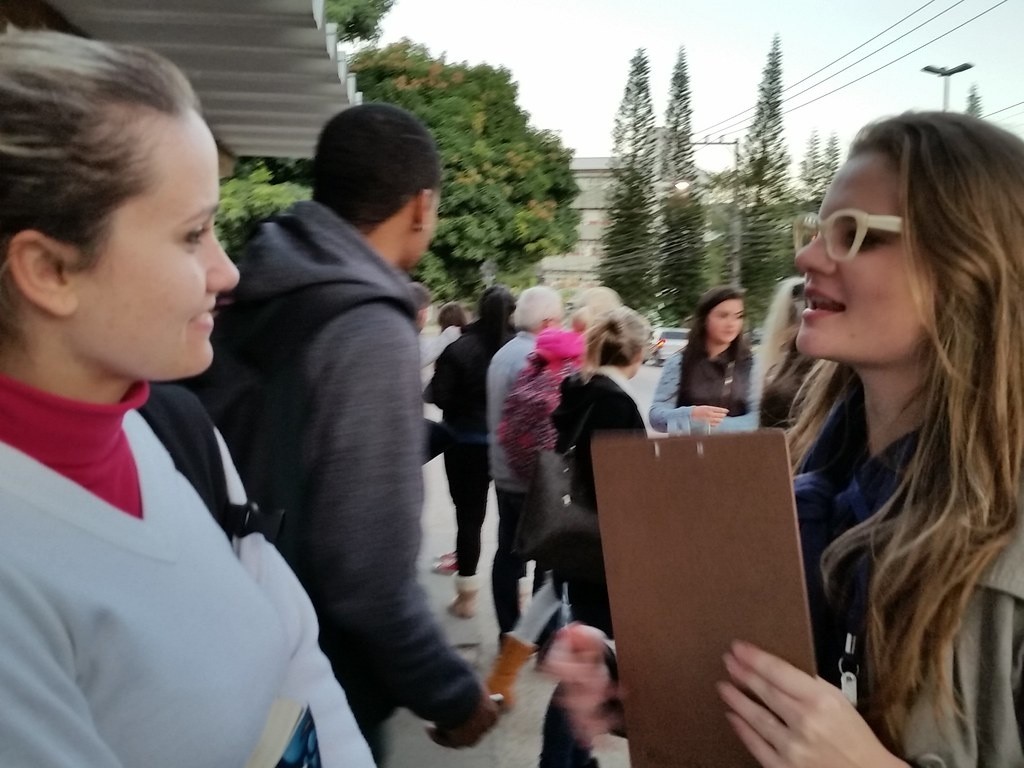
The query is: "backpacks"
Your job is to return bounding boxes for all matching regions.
[195,280,422,572]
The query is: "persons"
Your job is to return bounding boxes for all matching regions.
[208,103,511,768]
[430,272,805,714]
[546,111,1024,768]
[1,20,378,768]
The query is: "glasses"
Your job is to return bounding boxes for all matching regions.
[792,207,903,263]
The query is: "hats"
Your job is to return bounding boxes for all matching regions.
[696,285,738,318]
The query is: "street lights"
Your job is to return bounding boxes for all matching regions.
[920,63,974,110]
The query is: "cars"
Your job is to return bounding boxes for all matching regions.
[641,327,693,366]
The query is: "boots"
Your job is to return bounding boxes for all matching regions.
[481,629,539,714]
[446,575,479,621]
[515,576,533,616]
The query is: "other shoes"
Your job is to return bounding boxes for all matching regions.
[431,552,459,573]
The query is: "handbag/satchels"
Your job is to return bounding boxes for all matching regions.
[510,403,604,560]
